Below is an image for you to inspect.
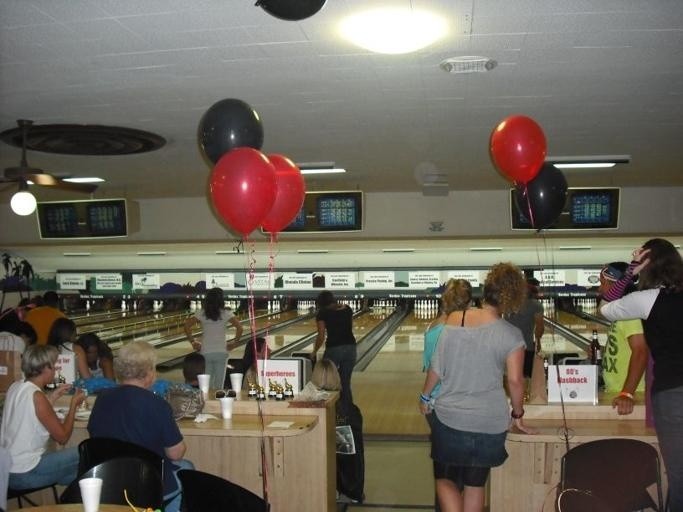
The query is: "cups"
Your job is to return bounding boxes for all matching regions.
[218,397,233,419]
[229,373,243,393]
[196,374,211,392]
[78,476,102,511]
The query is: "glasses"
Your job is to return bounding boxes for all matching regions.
[215,389,237,399]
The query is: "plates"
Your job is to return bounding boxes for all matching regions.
[74,411,90,421]
[52,406,68,419]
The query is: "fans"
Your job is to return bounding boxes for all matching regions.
[0,118,99,194]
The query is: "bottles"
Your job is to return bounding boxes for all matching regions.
[590,328,601,365]
[542,357,548,392]
[247,377,294,401]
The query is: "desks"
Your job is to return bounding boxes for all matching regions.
[490,377,667,511]
[8,390,340,512]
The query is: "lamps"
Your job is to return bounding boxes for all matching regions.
[10,182,37,216]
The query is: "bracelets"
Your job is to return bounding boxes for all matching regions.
[186,333,193,343]
[419,392,429,403]
[618,391,635,400]
[511,409,524,417]
[234,335,240,342]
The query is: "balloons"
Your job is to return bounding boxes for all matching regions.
[197,98,262,169]
[490,114,547,186]
[513,163,568,233]
[252,0,328,23]
[206,146,278,241]
[262,155,304,240]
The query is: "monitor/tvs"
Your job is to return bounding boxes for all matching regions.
[510,187,622,231]
[36,197,140,240]
[259,190,366,233]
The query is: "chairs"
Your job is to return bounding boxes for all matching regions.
[177,470,270,512]
[8,482,60,508]
[557,438,665,512]
[78,437,180,509]
[60,457,163,511]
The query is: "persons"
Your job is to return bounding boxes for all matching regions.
[1,344,84,489]
[599,261,650,416]
[421,278,470,510]
[307,290,357,402]
[417,260,539,511]
[1,291,116,390]
[88,341,195,512]
[184,286,242,389]
[181,353,205,388]
[308,357,366,503]
[229,337,272,391]
[505,283,544,405]
[597,237,682,511]
